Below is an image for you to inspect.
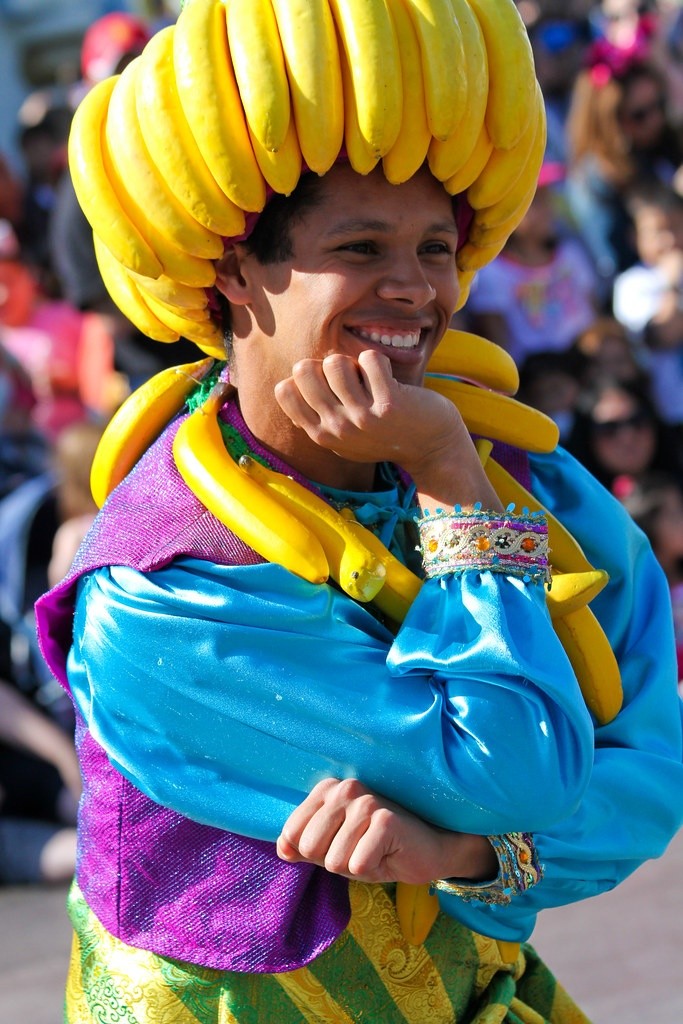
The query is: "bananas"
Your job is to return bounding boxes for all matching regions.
[91,327,622,728]
[67,0,546,359]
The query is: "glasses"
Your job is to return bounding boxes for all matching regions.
[593,410,648,443]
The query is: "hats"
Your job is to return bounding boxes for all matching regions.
[64,1,547,363]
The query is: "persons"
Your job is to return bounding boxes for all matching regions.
[0,0,210,883]
[449,0,683,688]
[35,0,683,1024]
